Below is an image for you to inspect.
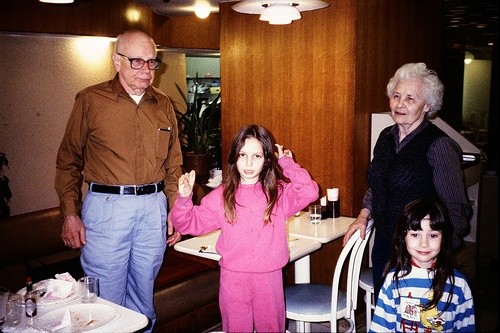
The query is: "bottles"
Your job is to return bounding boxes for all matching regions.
[24,277,37,317]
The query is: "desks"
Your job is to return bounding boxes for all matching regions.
[174,225,323,285]
[0,274,149,333]
[288,210,357,244]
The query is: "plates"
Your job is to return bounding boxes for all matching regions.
[16,280,85,308]
[38,303,119,333]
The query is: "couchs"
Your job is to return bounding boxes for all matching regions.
[0,197,220,333]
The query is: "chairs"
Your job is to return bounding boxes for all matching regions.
[284,218,379,333]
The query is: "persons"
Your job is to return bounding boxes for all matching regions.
[342,63,471,306]
[170,123,319,333]
[368,199,475,333]
[54,30,183,333]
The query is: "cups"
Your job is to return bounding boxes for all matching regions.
[78,276,98,303]
[309,200,339,225]
[0,289,22,327]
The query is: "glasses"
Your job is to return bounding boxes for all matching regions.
[118,53,161,70]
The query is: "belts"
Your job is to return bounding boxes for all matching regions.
[88,182,162,197]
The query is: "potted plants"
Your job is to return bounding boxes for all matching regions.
[169,71,221,184]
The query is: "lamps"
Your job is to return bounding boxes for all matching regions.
[234,0,330,26]
